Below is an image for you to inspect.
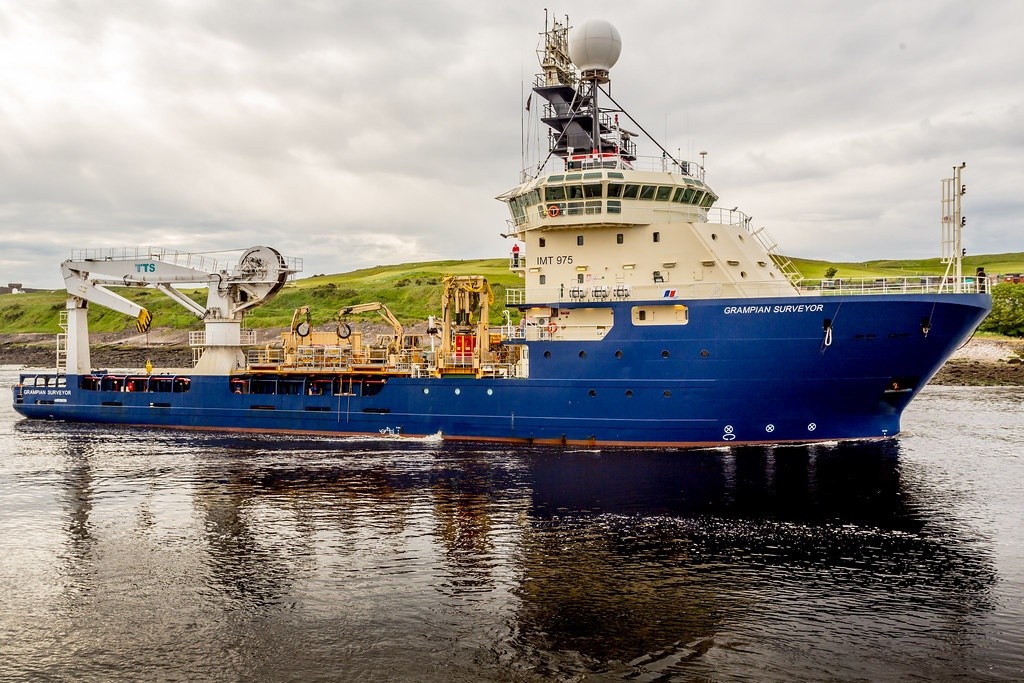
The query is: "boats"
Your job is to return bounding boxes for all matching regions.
[11,7,999,448]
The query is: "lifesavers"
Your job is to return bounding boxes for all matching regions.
[548,322,557,332]
[549,206,559,217]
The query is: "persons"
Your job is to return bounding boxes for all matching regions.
[512,244,520,267]
[519,314,526,338]
